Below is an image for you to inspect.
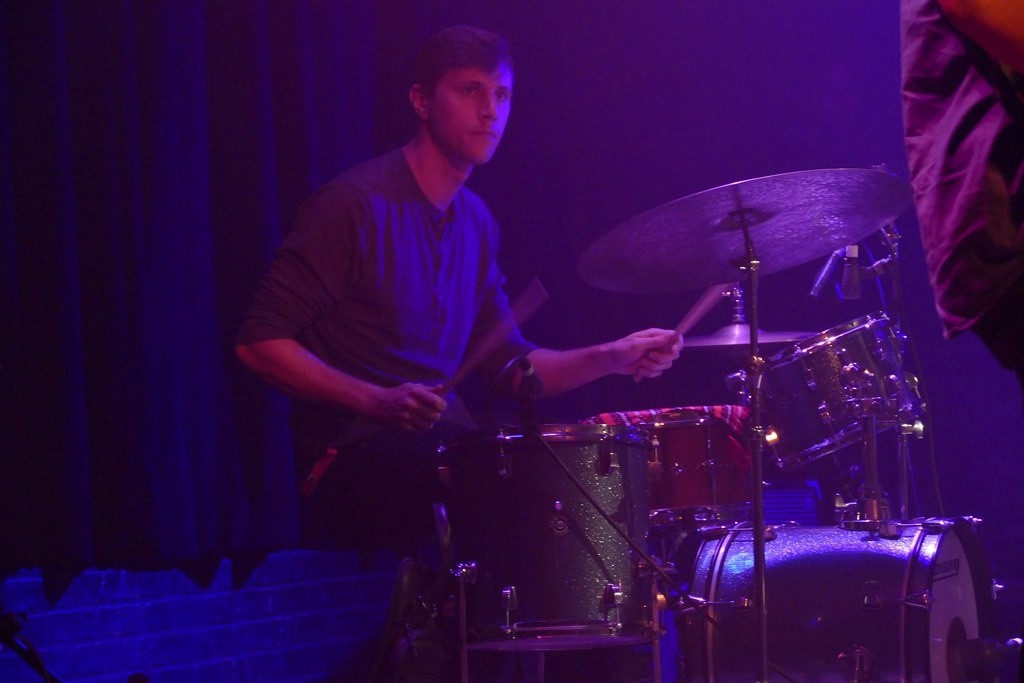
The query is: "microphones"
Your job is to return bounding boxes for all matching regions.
[519,359,545,402]
[810,247,844,298]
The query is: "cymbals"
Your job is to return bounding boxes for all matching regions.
[575,168,914,295]
[674,324,821,346]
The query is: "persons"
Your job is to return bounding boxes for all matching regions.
[233,27,685,681]
[899,0,1024,386]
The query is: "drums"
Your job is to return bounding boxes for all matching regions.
[585,405,759,543]
[679,518,978,682]
[727,308,930,467]
[436,423,663,635]
